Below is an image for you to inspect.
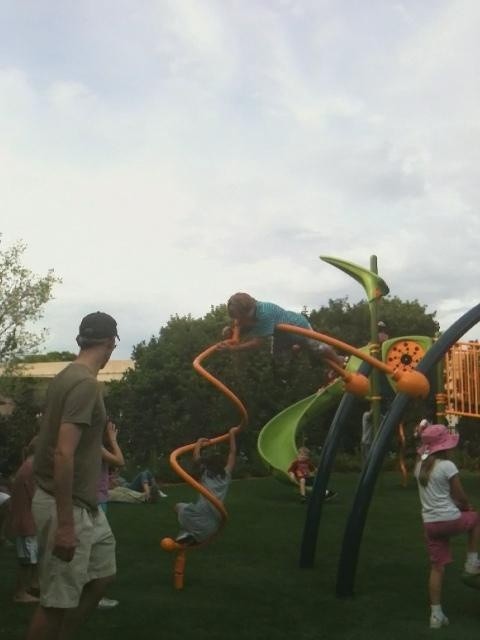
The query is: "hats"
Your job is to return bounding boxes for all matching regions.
[417,423,460,458]
[79,311,121,341]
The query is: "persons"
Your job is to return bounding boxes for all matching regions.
[361,411,385,457]
[217,293,345,379]
[287,446,337,504]
[10,421,169,611]
[27,311,120,640]
[415,424,480,629]
[173,425,241,544]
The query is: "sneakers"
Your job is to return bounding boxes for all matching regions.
[429,616,450,629]
[325,491,338,501]
[465,560,480,574]
[97,597,120,608]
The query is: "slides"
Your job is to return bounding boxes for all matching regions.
[257,345,372,493]
